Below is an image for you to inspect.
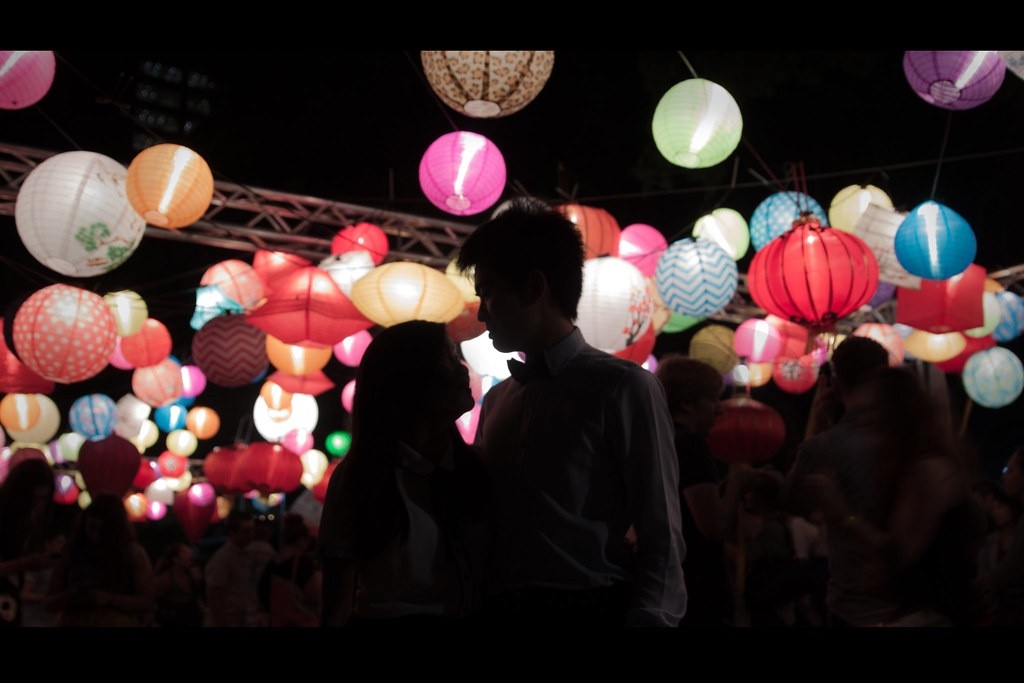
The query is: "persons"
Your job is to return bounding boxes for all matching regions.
[0,194,1024,683]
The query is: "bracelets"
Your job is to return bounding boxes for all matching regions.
[833,514,857,530]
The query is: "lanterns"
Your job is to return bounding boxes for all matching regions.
[0,50,1024,543]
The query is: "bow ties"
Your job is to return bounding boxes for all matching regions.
[507,359,537,385]
